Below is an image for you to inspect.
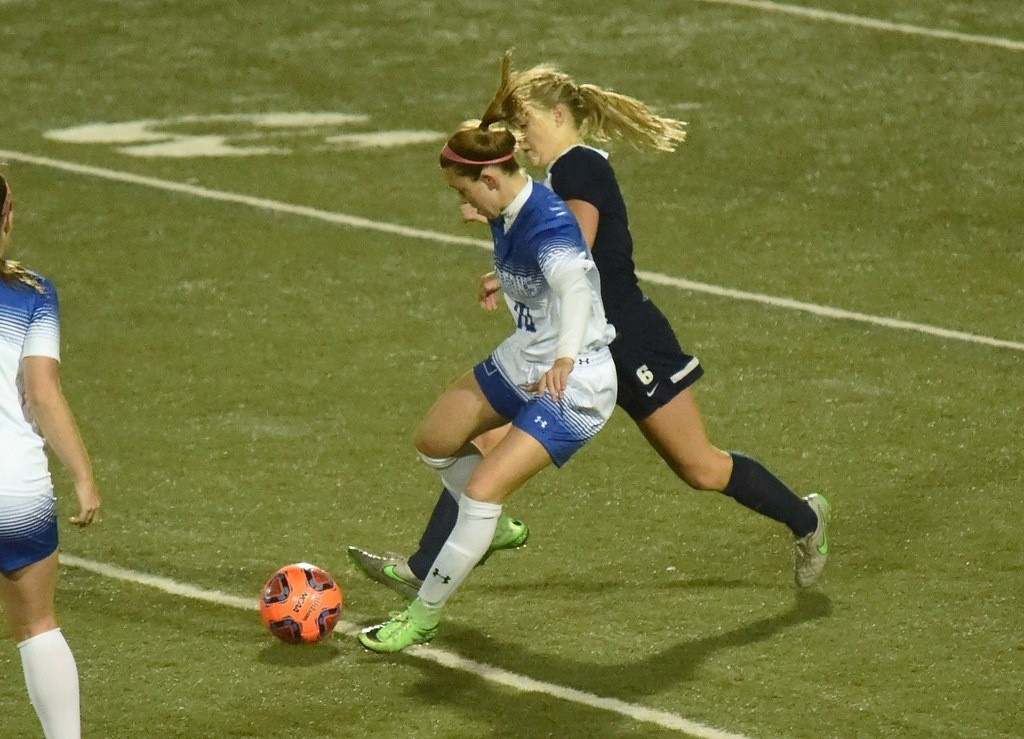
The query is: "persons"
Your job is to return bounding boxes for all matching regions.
[347,71,832,604]
[0,175,101,739]
[357,57,619,651]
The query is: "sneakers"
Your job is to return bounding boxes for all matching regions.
[475,513,529,570]
[358,604,439,654]
[793,493,832,588]
[347,546,421,600]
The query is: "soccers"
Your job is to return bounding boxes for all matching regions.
[259,561,344,645]
